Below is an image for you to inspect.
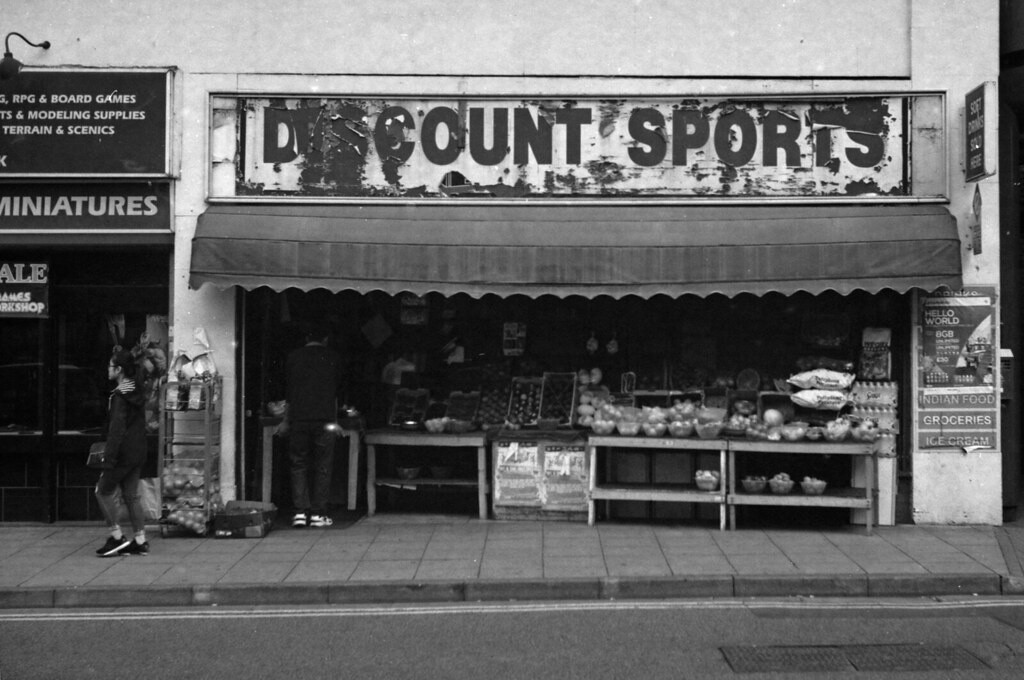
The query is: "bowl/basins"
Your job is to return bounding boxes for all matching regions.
[801,481,826,495]
[396,466,420,478]
[422,418,473,434]
[694,477,718,490]
[590,407,879,444]
[536,418,560,430]
[740,479,768,494]
[769,479,794,494]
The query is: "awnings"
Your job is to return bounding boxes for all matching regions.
[185,206,965,300]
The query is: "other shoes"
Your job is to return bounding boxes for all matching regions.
[310,522,334,527]
[291,513,307,520]
[96,535,130,556]
[292,520,307,526]
[310,514,332,522]
[117,538,150,556]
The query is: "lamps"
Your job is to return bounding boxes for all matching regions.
[0,32,50,80]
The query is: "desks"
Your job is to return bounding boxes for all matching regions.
[728,438,879,535]
[587,435,727,530]
[365,428,488,518]
[852,456,898,526]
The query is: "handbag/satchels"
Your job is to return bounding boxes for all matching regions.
[87,441,108,471]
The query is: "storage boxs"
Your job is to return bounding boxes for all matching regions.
[388,371,578,430]
[634,386,793,419]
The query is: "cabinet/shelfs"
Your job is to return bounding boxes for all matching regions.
[158,376,224,523]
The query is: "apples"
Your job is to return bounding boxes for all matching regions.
[479,381,573,425]
[694,470,720,491]
[743,473,827,496]
[587,400,875,439]
[162,450,225,535]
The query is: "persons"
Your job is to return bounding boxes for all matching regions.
[283,325,341,529]
[94,349,149,556]
